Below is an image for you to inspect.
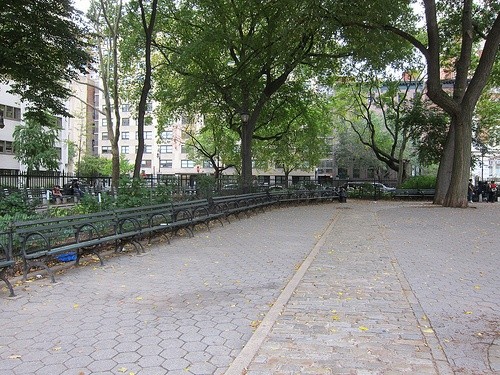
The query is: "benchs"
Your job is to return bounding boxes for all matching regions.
[0,190,340,298]
[393,189,435,203]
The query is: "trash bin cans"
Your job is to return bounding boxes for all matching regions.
[339,189,346,203]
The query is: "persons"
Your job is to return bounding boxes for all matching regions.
[52,185,65,204]
[468,179,500,203]
